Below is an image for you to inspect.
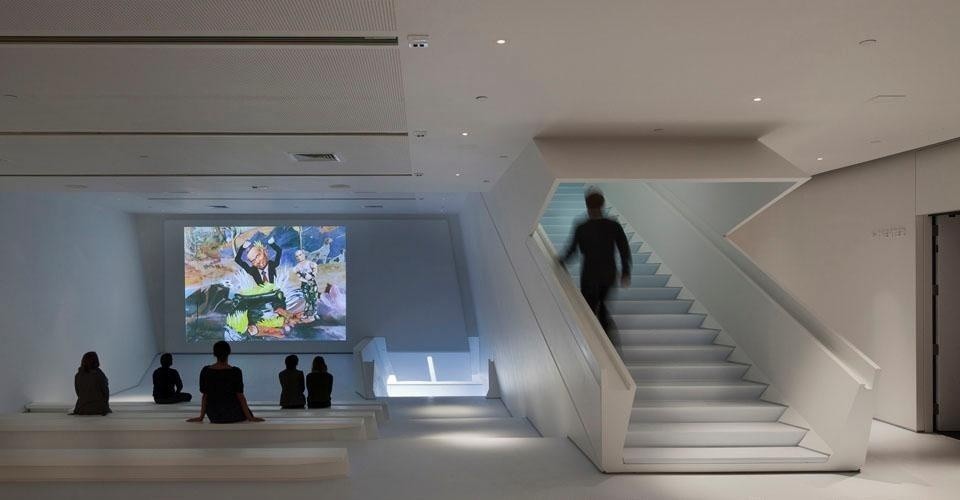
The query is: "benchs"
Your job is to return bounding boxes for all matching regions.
[3,395,391,483]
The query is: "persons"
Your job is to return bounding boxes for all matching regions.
[185,341,265,423]
[74,352,113,415]
[293,250,320,322]
[306,356,333,409]
[557,193,633,353]
[234,237,282,286]
[279,354,306,409]
[153,353,192,404]
